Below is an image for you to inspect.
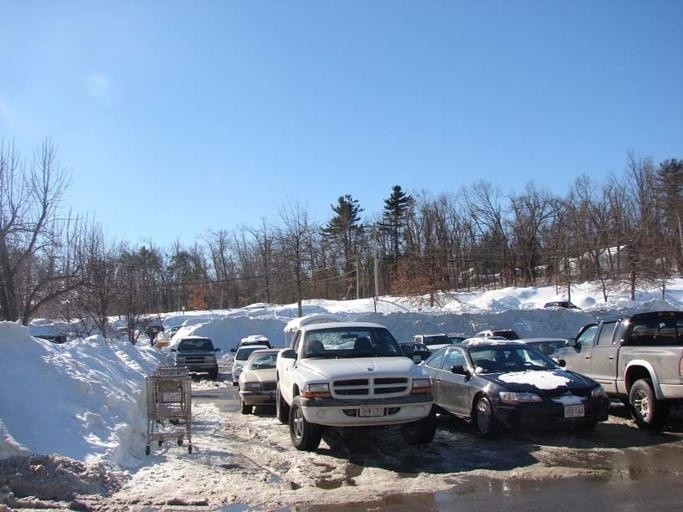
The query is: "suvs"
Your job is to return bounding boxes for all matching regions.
[171,337,220,379]
[275,320,436,452]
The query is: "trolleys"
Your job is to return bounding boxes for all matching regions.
[145,366,194,456]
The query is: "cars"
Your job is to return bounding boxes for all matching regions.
[422,341,610,441]
[234,349,282,414]
[509,338,568,368]
[236,335,275,351]
[231,345,274,387]
[396,329,519,363]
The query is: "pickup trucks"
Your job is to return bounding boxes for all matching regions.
[546,309,683,431]
